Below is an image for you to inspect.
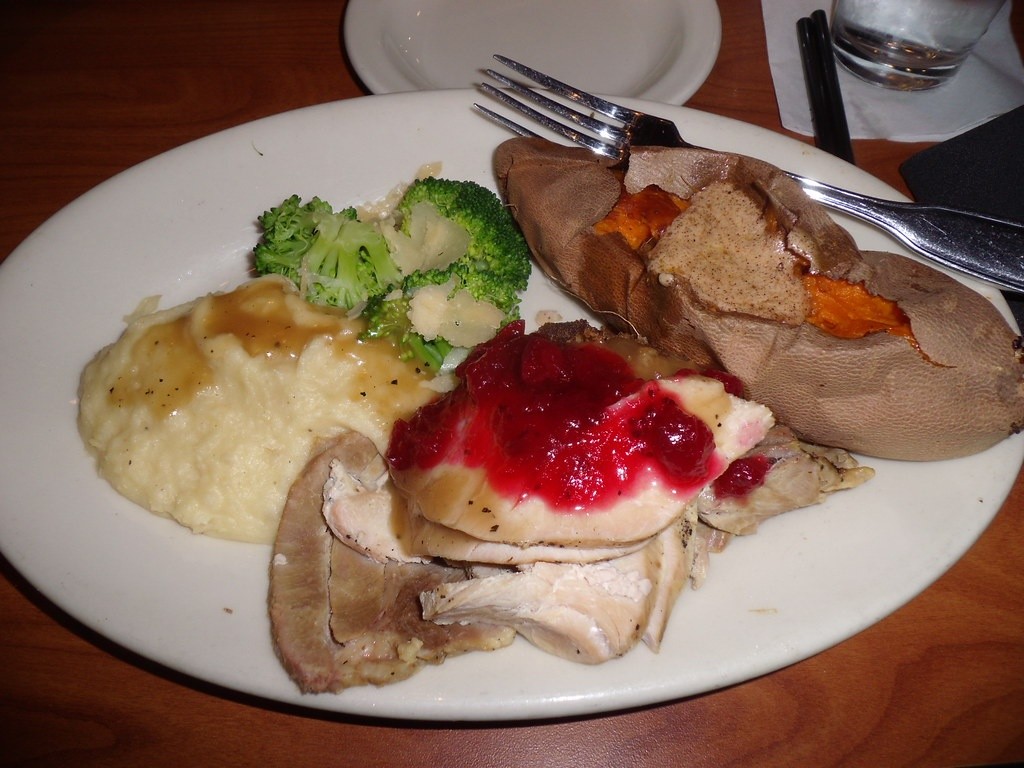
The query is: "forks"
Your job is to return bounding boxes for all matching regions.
[473,54,1024,294]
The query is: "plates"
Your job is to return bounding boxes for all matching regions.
[345,1,722,106]
[1,87,1024,721]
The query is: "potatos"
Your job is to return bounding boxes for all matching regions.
[493,136,1024,460]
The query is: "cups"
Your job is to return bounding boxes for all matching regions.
[830,0,1007,91]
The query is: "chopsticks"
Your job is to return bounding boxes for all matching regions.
[796,9,853,166]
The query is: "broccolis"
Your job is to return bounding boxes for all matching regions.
[253,176,531,379]
